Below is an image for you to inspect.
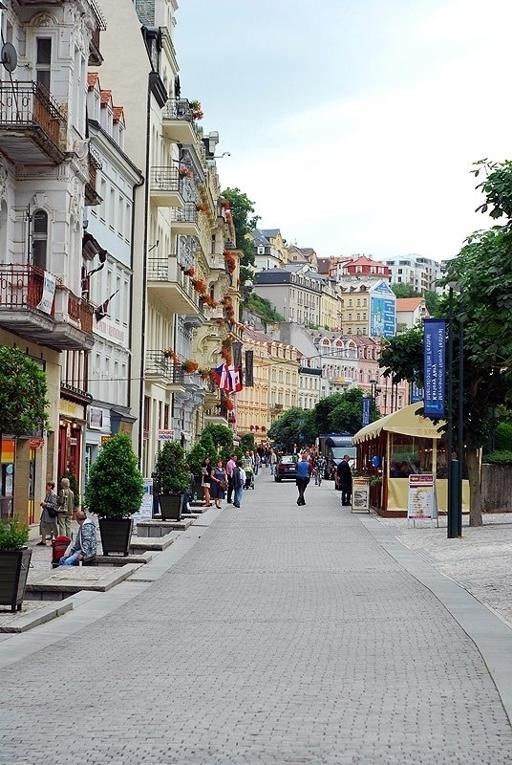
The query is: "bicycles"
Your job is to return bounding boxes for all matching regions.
[313,463,323,487]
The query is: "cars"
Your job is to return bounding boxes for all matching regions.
[273,455,299,484]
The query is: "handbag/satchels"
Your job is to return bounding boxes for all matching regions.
[217,479,227,491]
[47,500,58,516]
[55,494,69,512]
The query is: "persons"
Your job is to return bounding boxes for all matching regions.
[393,460,411,477]
[295,453,316,506]
[59,510,97,566]
[36,480,58,545]
[210,460,228,509]
[241,444,328,490]
[336,454,354,507]
[52,476,74,539]
[200,456,212,506]
[225,453,238,503]
[230,460,247,509]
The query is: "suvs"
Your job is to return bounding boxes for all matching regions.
[326,446,356,480]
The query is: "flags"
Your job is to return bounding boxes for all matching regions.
[212,363,243,397]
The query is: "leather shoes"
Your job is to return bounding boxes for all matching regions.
[203,504,211,507]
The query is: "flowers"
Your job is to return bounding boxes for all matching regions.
[172,95,238,414]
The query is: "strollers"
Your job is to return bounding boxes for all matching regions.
[243,465,255,491]
[49,531,78,570]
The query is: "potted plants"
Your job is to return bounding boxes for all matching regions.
[83,427,219,559]
[0,507,35,616]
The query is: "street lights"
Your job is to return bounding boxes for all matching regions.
[447,278,463,539]
[370,379,378,403]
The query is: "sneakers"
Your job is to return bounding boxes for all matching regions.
[297,500,306,506]
[215,499,222,509]
[37,541,46,547]
[342,500,352,506]
